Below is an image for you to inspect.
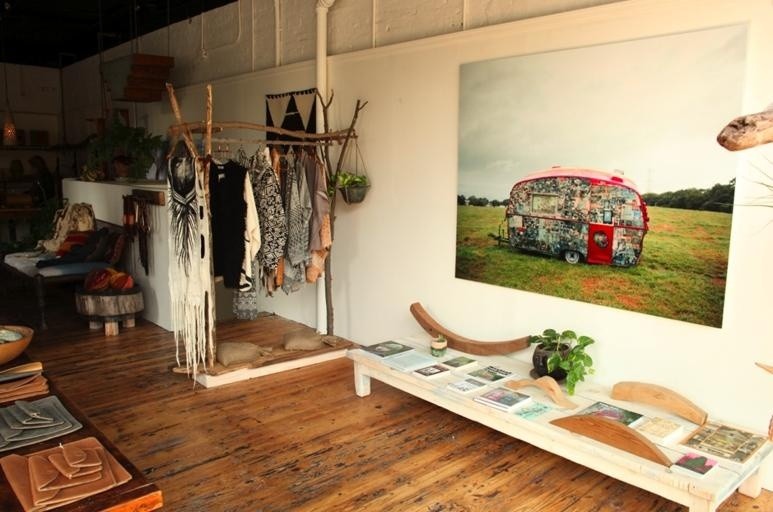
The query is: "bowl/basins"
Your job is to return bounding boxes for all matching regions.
[0,325,35,367]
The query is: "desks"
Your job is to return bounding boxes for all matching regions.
[349,337,771,512]
[0,348,164,512]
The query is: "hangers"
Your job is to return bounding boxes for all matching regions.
[212,137,309,160]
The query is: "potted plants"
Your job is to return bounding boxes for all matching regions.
[526,329,595,394]
[327,170,372,207]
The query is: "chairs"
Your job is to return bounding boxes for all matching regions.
[1,218,124,332]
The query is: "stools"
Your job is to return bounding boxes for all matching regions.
[72,285,144,337]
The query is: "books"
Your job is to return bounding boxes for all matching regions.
[362,340,516,394]
[671,421,767,475]
[475,386,532,413]
